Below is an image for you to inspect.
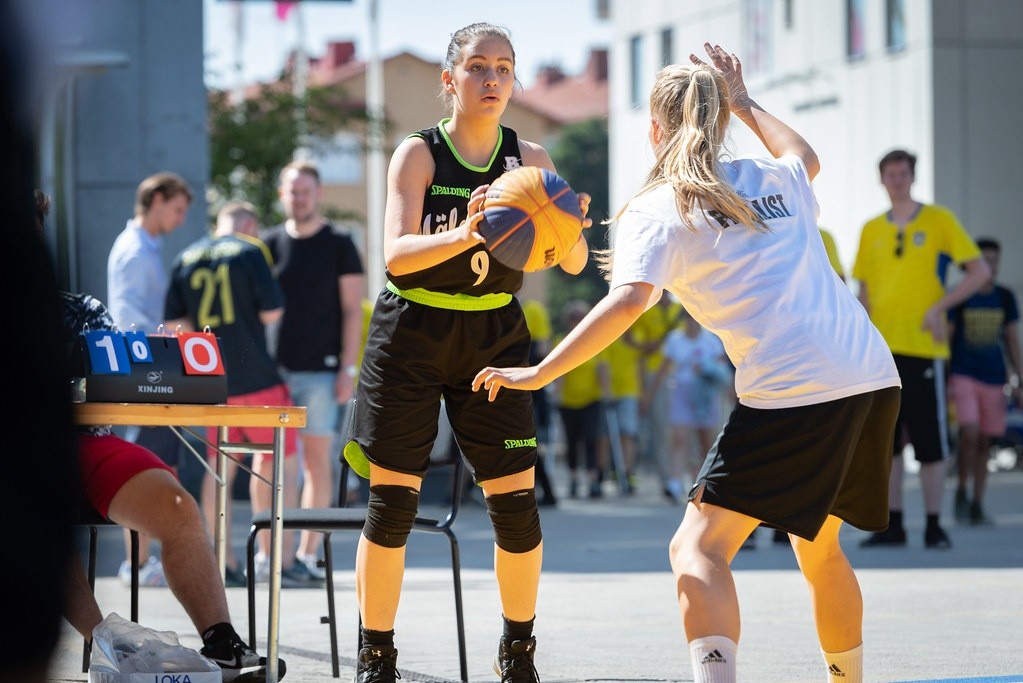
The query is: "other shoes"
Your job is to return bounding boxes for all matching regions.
[119,556,168,587]
[971,499,983,524]
[243,555,270,582]
[955,488,968,520]
[926,514,951,547]
[296,551,326,579]
[666,480,682,504]
[591,483,600,497]
[862,512,905,544]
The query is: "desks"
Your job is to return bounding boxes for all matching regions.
[76,401,307,683]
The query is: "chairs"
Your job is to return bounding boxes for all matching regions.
[248,393,471,683]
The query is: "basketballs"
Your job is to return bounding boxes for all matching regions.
[477,167,584,272]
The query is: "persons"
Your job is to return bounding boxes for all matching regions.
[356,21,588,683]
[26,183,287,683]
[107,161,362,588]
[850,150,990,551]
[942,236,1023,529]
[471,42,902,683]
[520,286,733,509]
[738,227,847,550]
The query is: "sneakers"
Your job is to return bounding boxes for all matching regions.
[353,648,401,683]
[493,635,540,683]
[200,632,286,683]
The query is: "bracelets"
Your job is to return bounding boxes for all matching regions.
[339,366,356,374]
